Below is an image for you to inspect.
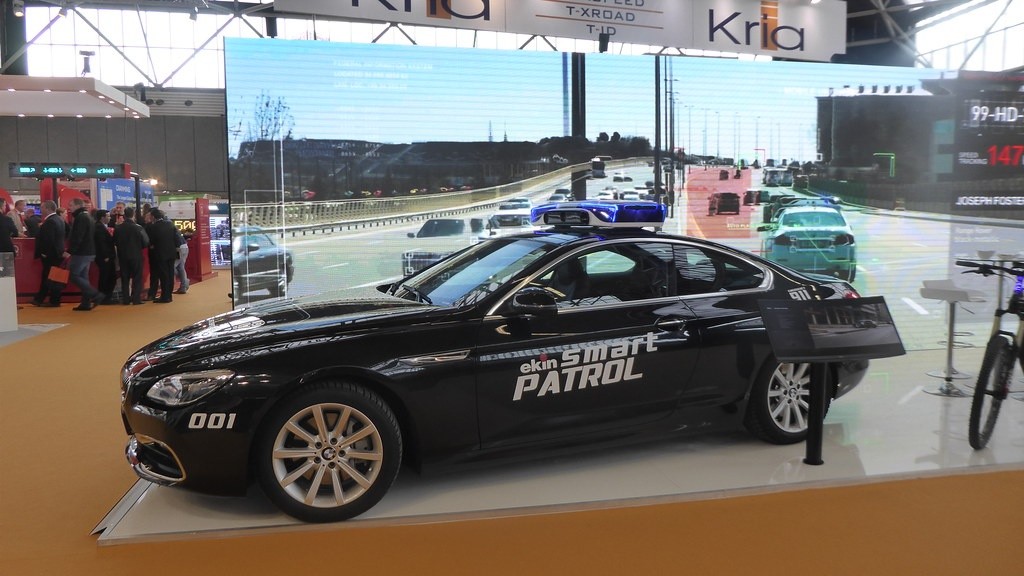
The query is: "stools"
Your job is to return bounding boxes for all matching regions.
[919,278,986,396]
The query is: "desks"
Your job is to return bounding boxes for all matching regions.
[958,252,1024,394]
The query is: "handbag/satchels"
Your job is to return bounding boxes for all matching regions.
[48,255,71,284]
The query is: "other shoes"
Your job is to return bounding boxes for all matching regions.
[142,294,156,301]
[90,293,105,309]
[123,302,130,305]
[39,300,60,307]
[173,287,186,294]
[29,299,40,306]
[153,297,172,303]
[133,301,144,304]
[73,304,91,311]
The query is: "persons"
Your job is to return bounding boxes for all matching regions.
[107,201,125,228]
[141,204,189,303]
[62,199,105,311]
[113,207,149,305]
[30,200,65,307]
[93,210,118,305]
[0,198,18,258]
[6,200,28,237]
[22,208,39,237]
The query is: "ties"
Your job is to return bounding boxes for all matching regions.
[19,214,23,224]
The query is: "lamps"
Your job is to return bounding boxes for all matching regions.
[189,3,198,21]
[13,0,25,17]
[57,0,68,17]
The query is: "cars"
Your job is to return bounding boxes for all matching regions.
[401,154,685,273]
[718,169,729,180]
[709,190,769,215]
[119,225,870,525]
[231,227,295,304]
[757,194,859,283]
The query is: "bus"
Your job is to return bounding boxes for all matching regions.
[763,164,803,187]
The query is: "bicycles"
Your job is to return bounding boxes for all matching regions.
[952,257,1024,448]
